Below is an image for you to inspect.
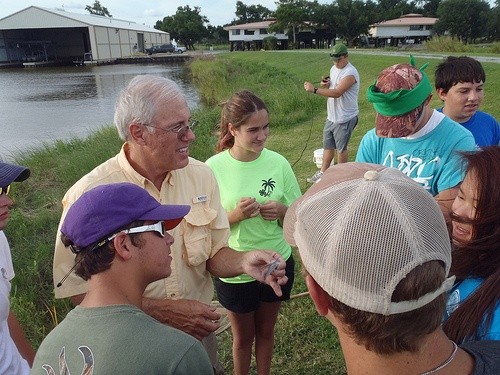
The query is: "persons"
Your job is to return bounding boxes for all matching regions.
[435,56,500,146]
[304,44,360,183]
[53,76,287,375]
[32,183,214,375]
[355,54,475,214]
[202,91,303,375]
[284,163,500,375]
[440,148,500,340]
[0,158,36,375]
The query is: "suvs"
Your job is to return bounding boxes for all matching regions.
[145,44,174,56]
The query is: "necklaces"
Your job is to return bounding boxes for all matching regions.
[421,341,458,375]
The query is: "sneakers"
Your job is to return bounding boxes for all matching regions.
[306,170,324,183]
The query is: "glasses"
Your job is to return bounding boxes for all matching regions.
[330,53,347,57]
[136,120,199,140]
[98,221,166,246]
[0,184,10,196]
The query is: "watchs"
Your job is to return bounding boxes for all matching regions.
[314,87,318,93]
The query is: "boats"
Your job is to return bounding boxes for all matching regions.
[16,39,64,66]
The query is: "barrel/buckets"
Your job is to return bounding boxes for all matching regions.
[314,149,334,169]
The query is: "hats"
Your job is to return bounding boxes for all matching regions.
[375,63,425,138]
[330,44,348,60]
[60,182,191,254]
[0,159,30,187]
[283,162,456,315]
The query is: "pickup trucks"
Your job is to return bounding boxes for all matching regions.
[174,47,186,54]
